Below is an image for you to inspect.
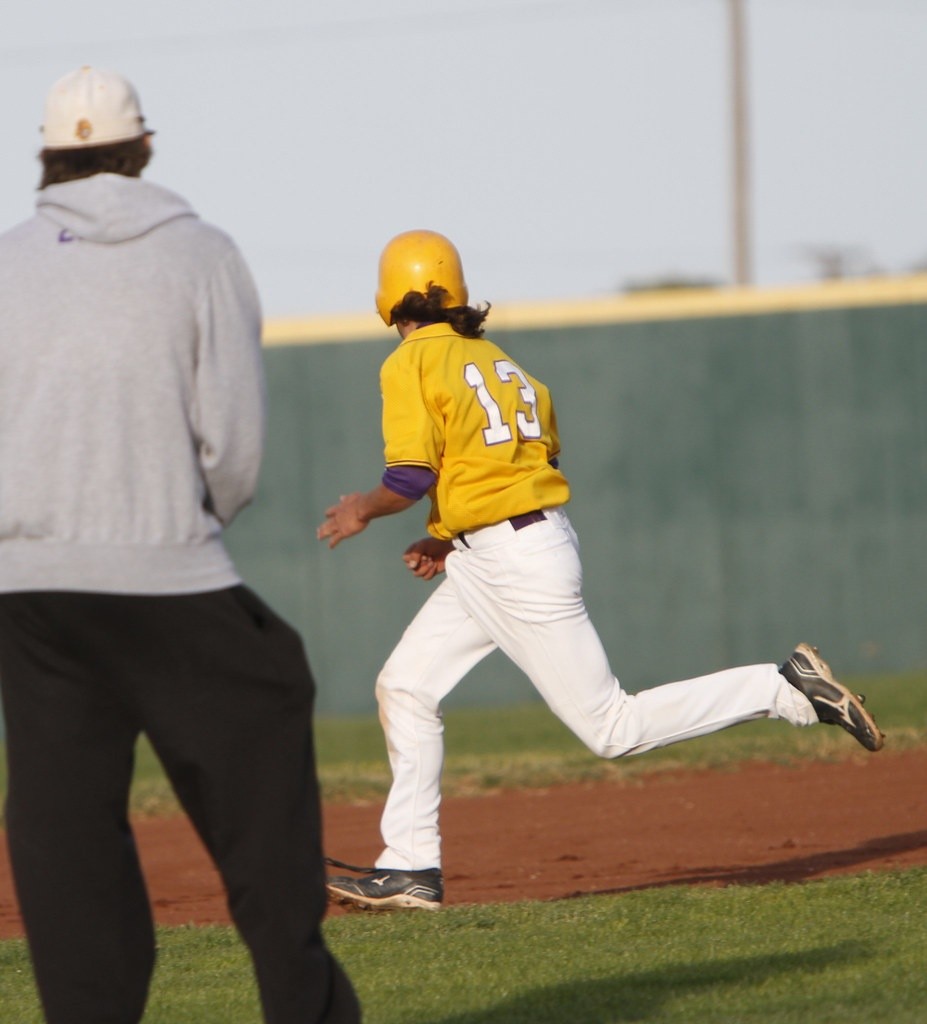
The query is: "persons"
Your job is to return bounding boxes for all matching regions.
[315,229,883,912]
[0,66,363,1024]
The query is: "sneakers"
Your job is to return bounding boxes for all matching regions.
[778,642,886,752]
[323,857,443,911]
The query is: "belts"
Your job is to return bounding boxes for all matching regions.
[457,508,546,549]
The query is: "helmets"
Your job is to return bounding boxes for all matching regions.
[376,230,468,328]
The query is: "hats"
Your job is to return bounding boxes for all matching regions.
[40,65,145,150]
